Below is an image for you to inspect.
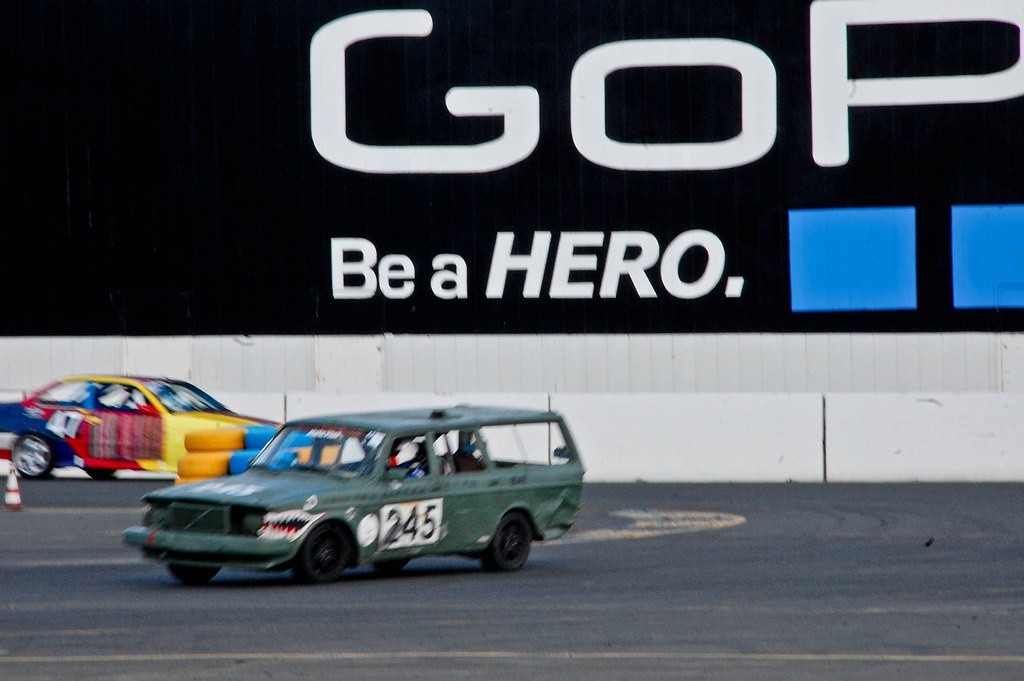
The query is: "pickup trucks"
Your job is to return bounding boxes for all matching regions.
[121,403,586,581]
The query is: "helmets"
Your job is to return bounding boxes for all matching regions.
[387,438,419,466]
[144,382,178,415]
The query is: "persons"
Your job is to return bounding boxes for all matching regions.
[385,438,426,480]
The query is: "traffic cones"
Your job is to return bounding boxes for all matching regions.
[1,469,26,513]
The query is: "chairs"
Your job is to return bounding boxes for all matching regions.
[453,450,482,471]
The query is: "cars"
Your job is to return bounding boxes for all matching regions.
[0,374,288,479]
